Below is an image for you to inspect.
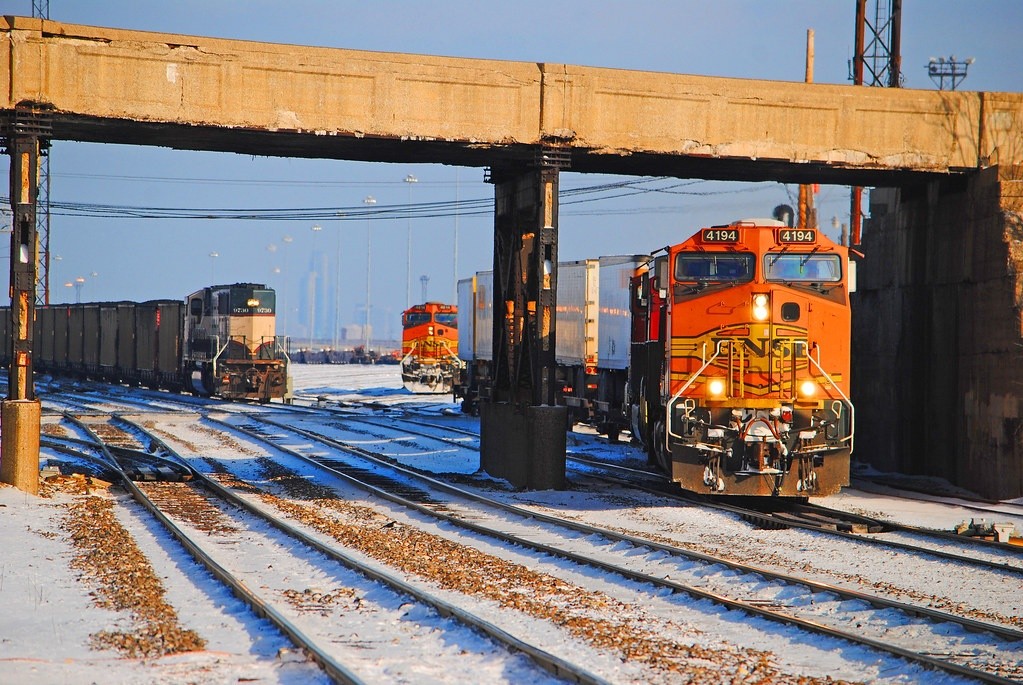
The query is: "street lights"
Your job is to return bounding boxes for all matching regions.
[208,208,348,351]
[362,195,374,352]
[401,172,417,307]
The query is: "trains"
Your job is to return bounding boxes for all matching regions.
[400,300,460,382]
[0,282,288,405]
[446,215,858,503]
